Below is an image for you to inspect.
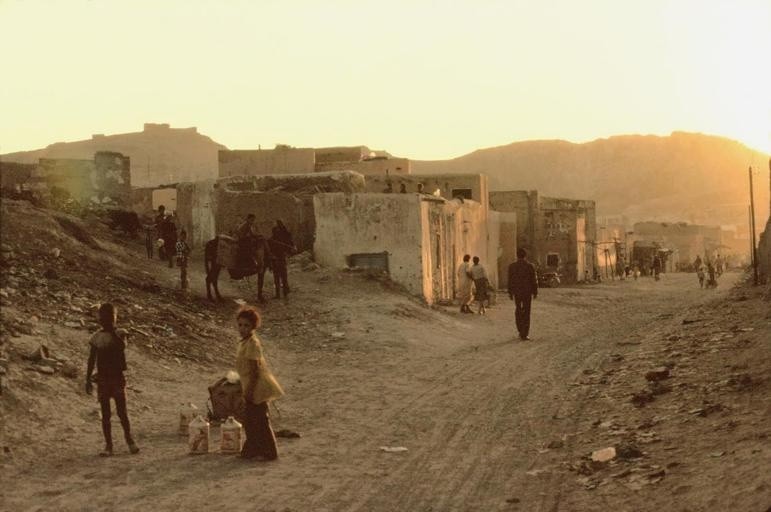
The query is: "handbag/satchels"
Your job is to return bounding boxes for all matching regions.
[242,369,286,403]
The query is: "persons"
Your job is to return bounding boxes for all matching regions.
[508,247,537,340]
[616,253,662,282]
[236,308,284,462]
[145,205,176,268]
[694,254,723,289]
[85,303,140,457]
[268,219,296,298]
[458,255,491,315]
[235,214,261,266]
[176,228,191,291]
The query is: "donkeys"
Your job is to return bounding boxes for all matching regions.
[205,220,298,304]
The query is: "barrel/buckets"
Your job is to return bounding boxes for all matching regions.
[187,414,209,454]
[220,416,241,455]
[179,401,198,435]
